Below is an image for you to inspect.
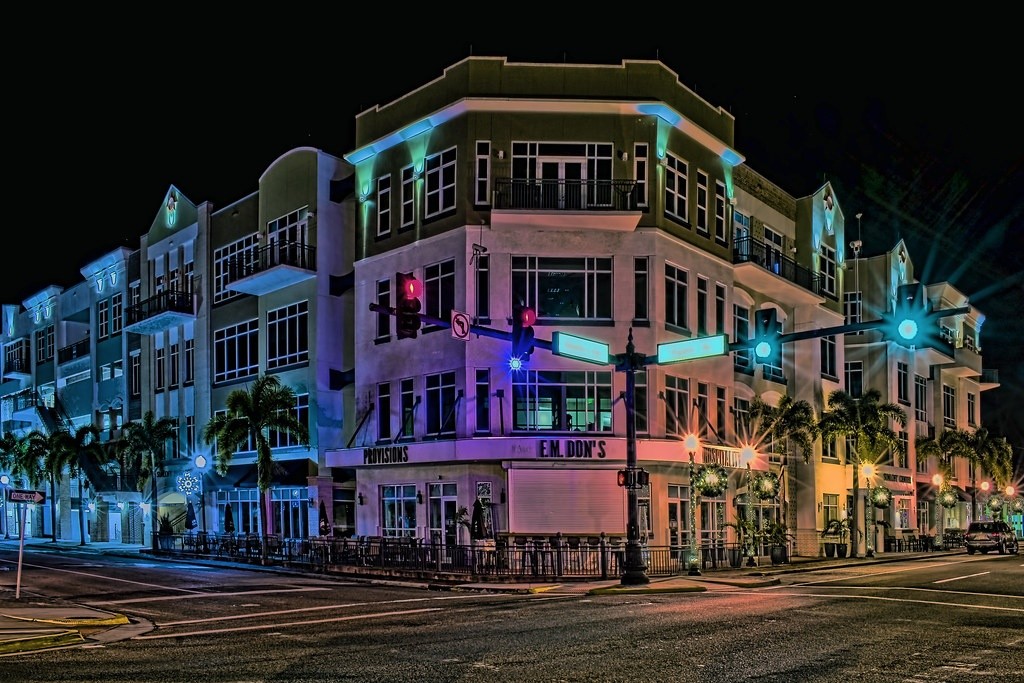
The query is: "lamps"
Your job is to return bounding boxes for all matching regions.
[660,158,668,166]
[413,163,423,180]
[500,488,506,504]
[359,184,370,202]
[729,198,737,205]
[358,492,363,506]
[308,497,314,508]
[416,490,423,504]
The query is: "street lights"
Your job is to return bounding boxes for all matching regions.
[741,443,758,566]
[0,475,10,539]
[195,456,209,553]
[933,473,945,550]
[685,432,702,575]
[1005,486,1014,523]
[861,462,876,557]
[981,482,991,516]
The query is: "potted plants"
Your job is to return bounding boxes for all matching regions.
[766,520,795,566]
[821,518,864,558]
[156,512,174,551]
[450,505,471,568]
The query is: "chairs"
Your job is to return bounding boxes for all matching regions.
[183,531,424,568]
[896,534,959,552]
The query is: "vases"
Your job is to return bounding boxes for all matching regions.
[824,543,836,558]
[728,549,744,568]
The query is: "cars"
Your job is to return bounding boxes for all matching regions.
[965,519,1019,556]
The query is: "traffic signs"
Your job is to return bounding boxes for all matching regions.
[8,489,46,504]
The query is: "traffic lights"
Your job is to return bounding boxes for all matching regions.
[638,471,650,486]
[512,305,535,362]
[895,283,924,346]
[617,471,632,485]
[754,307,780,363]
[395,272,422,338]
